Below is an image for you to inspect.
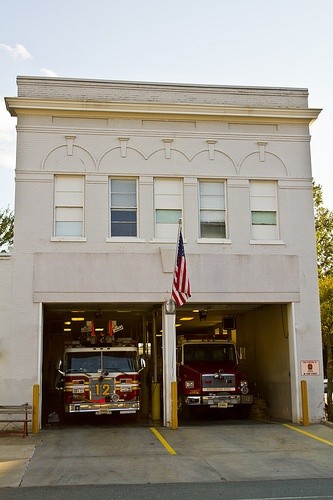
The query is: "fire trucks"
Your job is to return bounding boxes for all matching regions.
[56,320,146,421]
[176,333,254,415]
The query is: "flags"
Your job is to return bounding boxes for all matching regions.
[171,228,192,310]
